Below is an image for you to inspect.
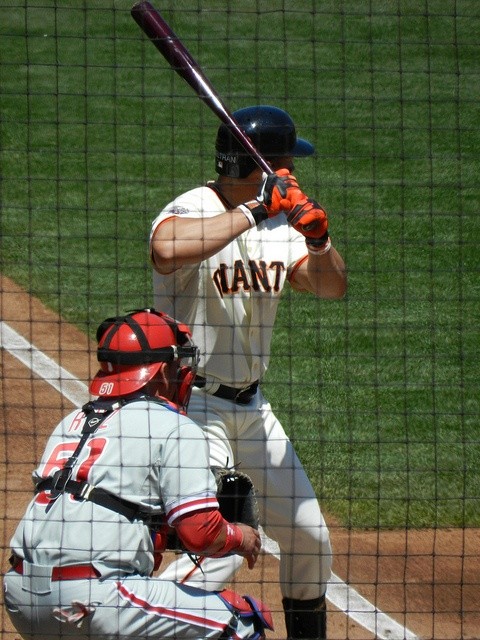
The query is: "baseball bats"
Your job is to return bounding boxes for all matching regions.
[131,0,286,198]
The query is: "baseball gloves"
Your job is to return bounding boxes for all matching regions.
[161,466,259,550]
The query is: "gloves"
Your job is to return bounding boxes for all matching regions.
[237,168,298,228]
[279,187,331,255]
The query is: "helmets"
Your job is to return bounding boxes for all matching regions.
[89,307,200,416]
[216,105,314,180]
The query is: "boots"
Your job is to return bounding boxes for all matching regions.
[282,593,326,639]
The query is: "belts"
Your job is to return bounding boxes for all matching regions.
[194,375,258,405]
[13,556,100,581]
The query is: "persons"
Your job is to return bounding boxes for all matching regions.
[149,105,347,639]
[4,308,274,639]
